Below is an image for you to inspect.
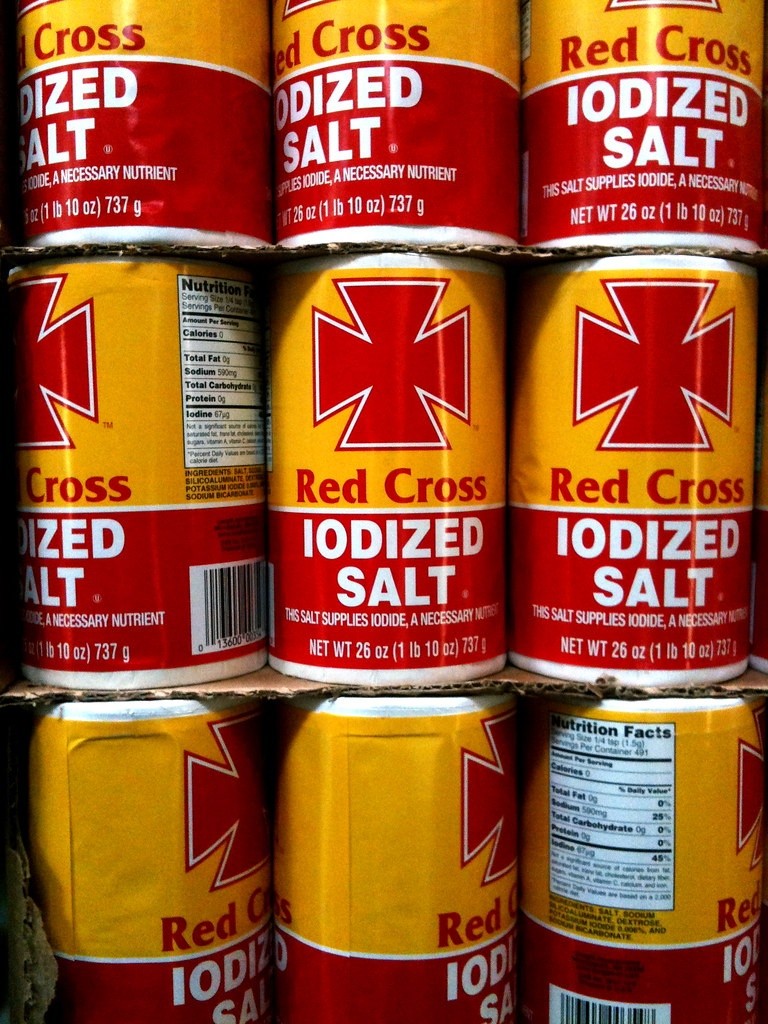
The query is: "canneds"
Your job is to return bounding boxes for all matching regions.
[9,0,768,1024]
